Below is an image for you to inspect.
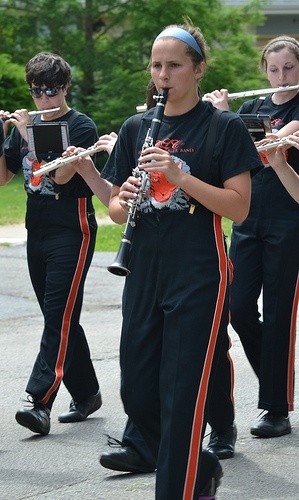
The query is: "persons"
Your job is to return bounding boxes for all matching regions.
[100,17,265,499]
[0,49,103,436]
[62,80,266,500]
[199,36,299,437]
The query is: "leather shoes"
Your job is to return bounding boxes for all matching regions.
[203,423,237,459]
[99,434,155,473]
[250,410,291,437]
[15,395,50,434]
[57,391,102,423]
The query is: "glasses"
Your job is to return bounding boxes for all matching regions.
[28,82,65,98]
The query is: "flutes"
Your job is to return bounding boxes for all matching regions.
[255,133,298,154]
[106,86,171,278]
[0,107,61,123]
[134,83,298,113]
[32,145,98,178]
